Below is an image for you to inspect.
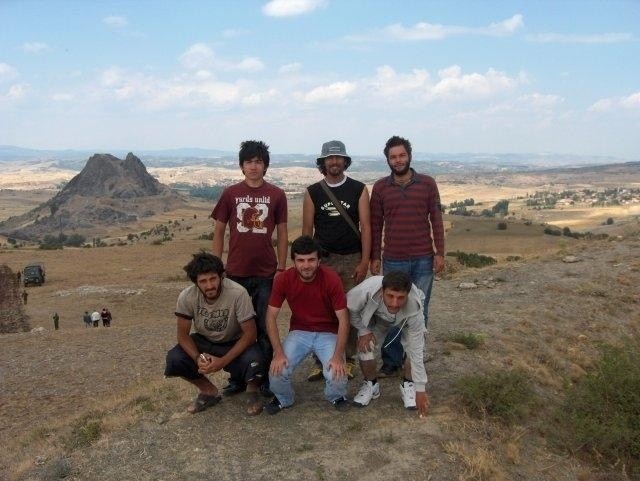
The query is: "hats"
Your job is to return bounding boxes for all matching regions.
[316,140,351,165]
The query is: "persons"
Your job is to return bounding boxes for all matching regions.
[101,309,108,327]
[53,313,60,330]
[21,290,29,305]
[163,250,270,418]
[265,236,350,412]
[300,140,371,381]
[105,308,112,327]
[83,312,92,328]
[369,135,445,379]
[209,141,288,400]
[342,271,430,419]
[16,270,22,286]
[91,309,101,328]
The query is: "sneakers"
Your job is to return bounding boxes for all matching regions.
[345,362,360,379]
[266,396,291,415]
[332,396,351,412]
[221,382,244,396]
[307,367,323,381]
[378,366,402,378]
[352,381,380,408]
[398,381,417,410]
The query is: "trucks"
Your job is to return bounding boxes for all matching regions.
[22,263,46,285]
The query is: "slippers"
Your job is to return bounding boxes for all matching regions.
[187,394,221,413]
[245,391,264,416]
